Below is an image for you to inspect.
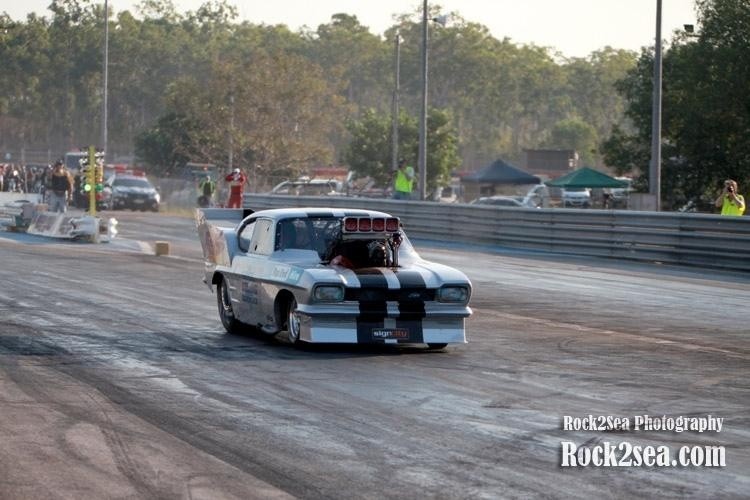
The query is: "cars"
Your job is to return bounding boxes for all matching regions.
[103,164,161,212]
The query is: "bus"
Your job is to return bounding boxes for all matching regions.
[63,151,106,180]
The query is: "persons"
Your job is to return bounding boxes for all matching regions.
[224,167,247,209]
[714,179,746,217]
[388,158,415,200]
[199,175,215,207]
[47,158,73,213]
[0,156,88,209]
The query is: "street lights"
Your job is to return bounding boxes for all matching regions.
[419,0,439,200]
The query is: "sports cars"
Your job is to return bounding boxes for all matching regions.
[196,207,473,348]
